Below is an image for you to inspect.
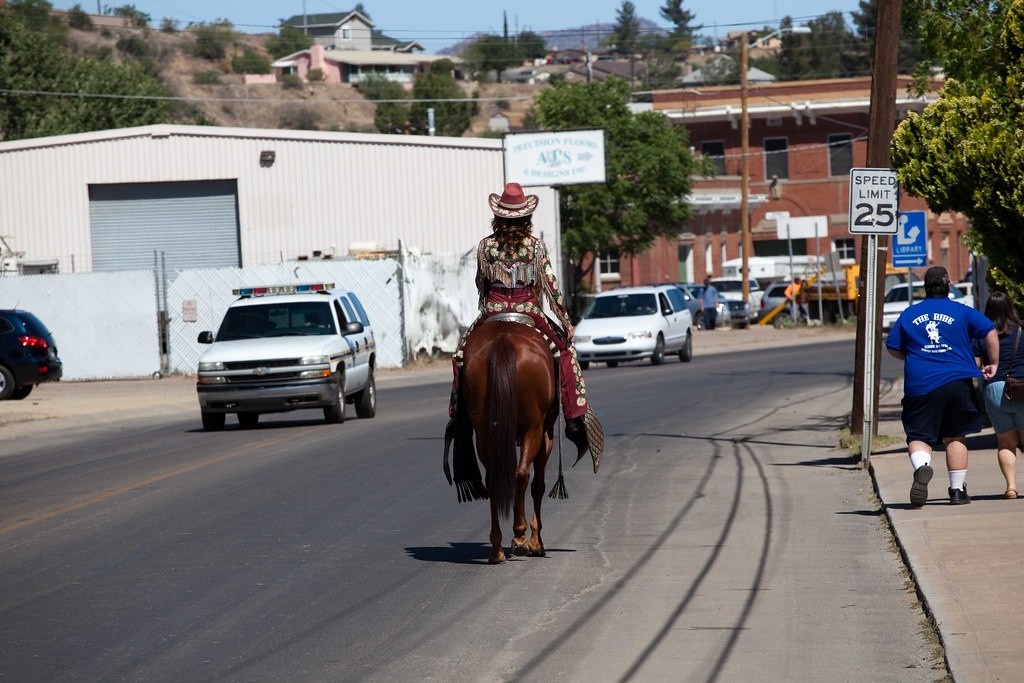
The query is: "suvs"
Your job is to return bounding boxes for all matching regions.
[0,309,64,399]
[196,281,378,432]
[570,284,694,372]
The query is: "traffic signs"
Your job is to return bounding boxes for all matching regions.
[892,209,928,267]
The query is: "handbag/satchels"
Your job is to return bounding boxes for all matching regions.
[1003,376,1024,401]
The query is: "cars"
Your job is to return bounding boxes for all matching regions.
[759,281,810,328]
[669,283,731,329]
[954,282,975,310]
[881,279,965,337]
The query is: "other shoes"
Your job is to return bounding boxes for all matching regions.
[567,418,585,434]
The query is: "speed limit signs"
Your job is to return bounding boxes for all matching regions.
[846,166,901,235]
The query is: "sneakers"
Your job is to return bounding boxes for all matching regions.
[948,482,971,506]
[910,463,933,508]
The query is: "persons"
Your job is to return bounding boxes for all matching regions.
[449,182,597,434]
[701,278,719,330]
[885,265,999,504]
[784,276,805,321]
[972,291,1024,499]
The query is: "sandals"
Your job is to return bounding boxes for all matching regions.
[1005,489,1019,498]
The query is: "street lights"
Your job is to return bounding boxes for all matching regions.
[741,25,813,325]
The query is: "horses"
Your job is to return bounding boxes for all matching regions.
[462,319,559,564]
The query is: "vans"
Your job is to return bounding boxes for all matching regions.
[711,276,764,330]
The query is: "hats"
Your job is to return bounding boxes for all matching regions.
[488,182,539,218]
[923,266,950,287]
